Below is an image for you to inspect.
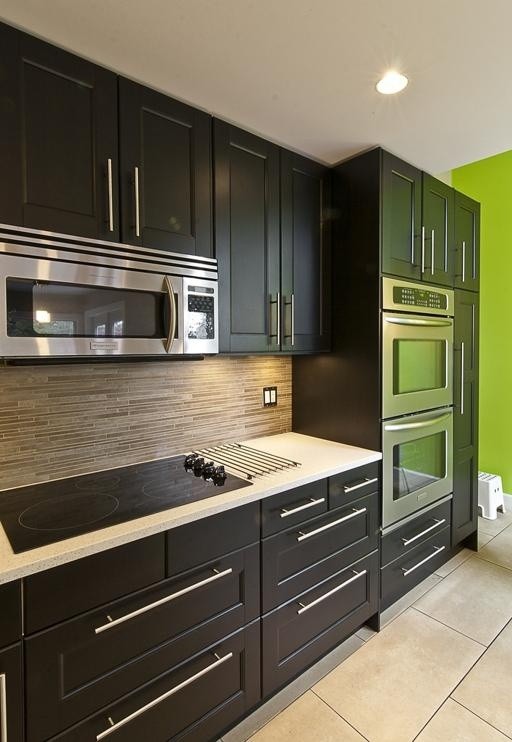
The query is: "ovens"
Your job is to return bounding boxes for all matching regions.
[377,276,458,420]
[379,407,454,528]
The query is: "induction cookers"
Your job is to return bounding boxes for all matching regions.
[0,447,254,556]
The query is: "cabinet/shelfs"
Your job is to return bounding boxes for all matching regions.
[0,18,218,262]
[453,288,480,553]
[1,499,261,742]
[258,461,382,700]
[213,113,336,358]
[330,146,484,294]
[380,497,454,635]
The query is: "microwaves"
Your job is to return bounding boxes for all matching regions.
[0,251,222,367]
